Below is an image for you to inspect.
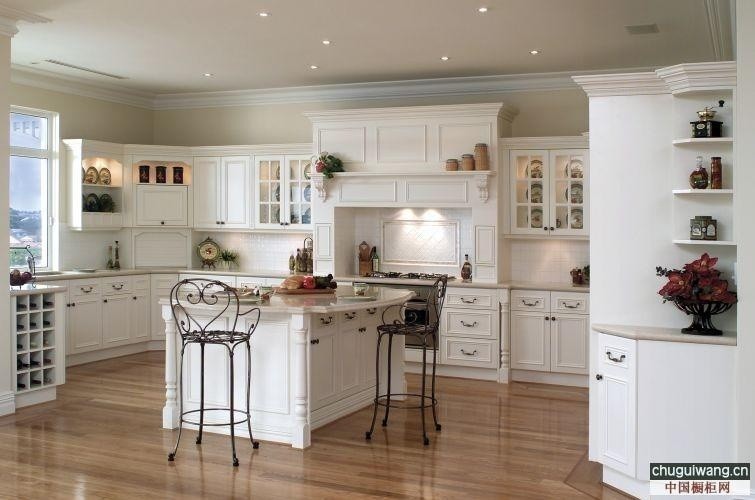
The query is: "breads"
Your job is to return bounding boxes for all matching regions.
[280,275,305,290]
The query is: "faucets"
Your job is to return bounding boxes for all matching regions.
[26,257,34,274]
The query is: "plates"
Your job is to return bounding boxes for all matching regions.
[99,195,113,212]
[566,209,583,229]
[275,166,279,179]
[304,185,312,202]
[86,167,99,184]
[304,165,311,180]
[83,195,86,211]
[100,167,112,185]
[566,160,584,178]
[197,240,220,263]
[338,294,377,301]
[526,183,543,203]
[87,194,101,212]
[526,209,543,228]
[565,183,583,203]
[276,186,280,201]
[526,160,543,178]
[305,209,311,223]
[275,209,279,223]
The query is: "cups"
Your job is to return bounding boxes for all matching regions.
[259,286,276,300]
[355,283,369,297]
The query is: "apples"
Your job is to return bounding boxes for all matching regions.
[10,268,21,286]
[21,271,32,281]
[302,276,316,289]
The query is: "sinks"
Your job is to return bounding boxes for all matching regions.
[29,271,63,277]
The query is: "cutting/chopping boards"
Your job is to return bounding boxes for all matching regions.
[274,287,335,295]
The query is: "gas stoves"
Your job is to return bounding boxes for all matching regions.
[373,271,454,281]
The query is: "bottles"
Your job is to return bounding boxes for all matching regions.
[106,246,114,269]
[570,268,583,285]
[689,156,708,189]
[289,241,313,273]
[709,156,723,190]
[115,241,120,269]
[370,247,379,272]
[358,241,372,276]
[462,255,473,281]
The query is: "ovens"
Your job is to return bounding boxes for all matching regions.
[368,283,438,350]
